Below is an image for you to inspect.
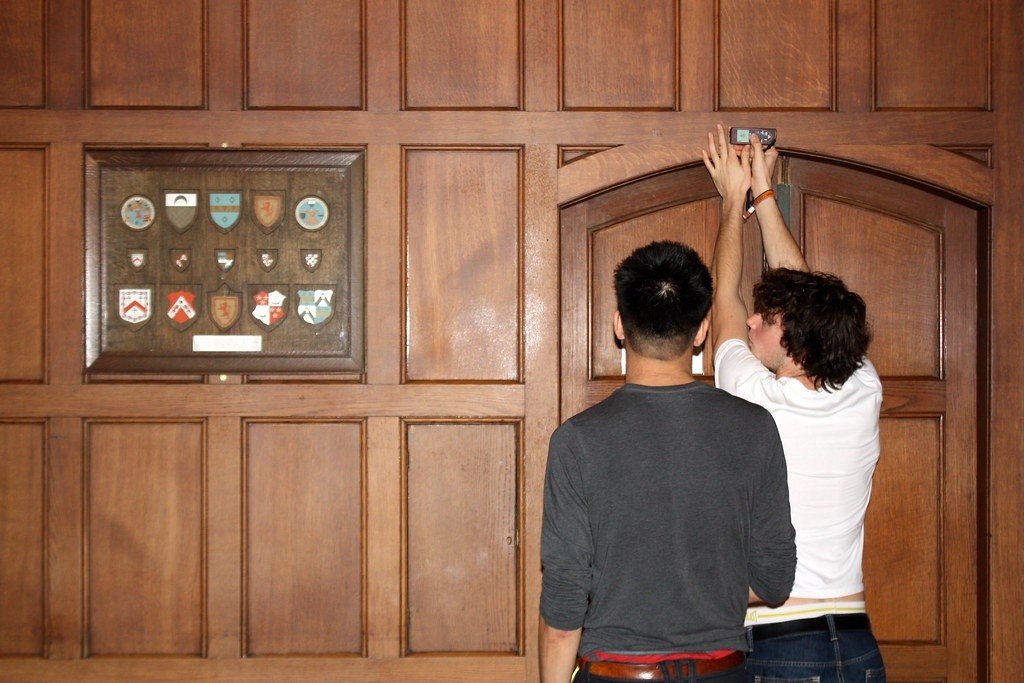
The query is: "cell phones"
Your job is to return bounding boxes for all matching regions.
[730,127,777,145]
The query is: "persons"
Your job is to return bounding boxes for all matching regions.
[701,122,891,683]
[533,241,798,683]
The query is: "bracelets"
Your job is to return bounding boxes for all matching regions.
[742,190,777,218]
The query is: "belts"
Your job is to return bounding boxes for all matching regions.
[576,651,744,680]
[751,615,871,641]
